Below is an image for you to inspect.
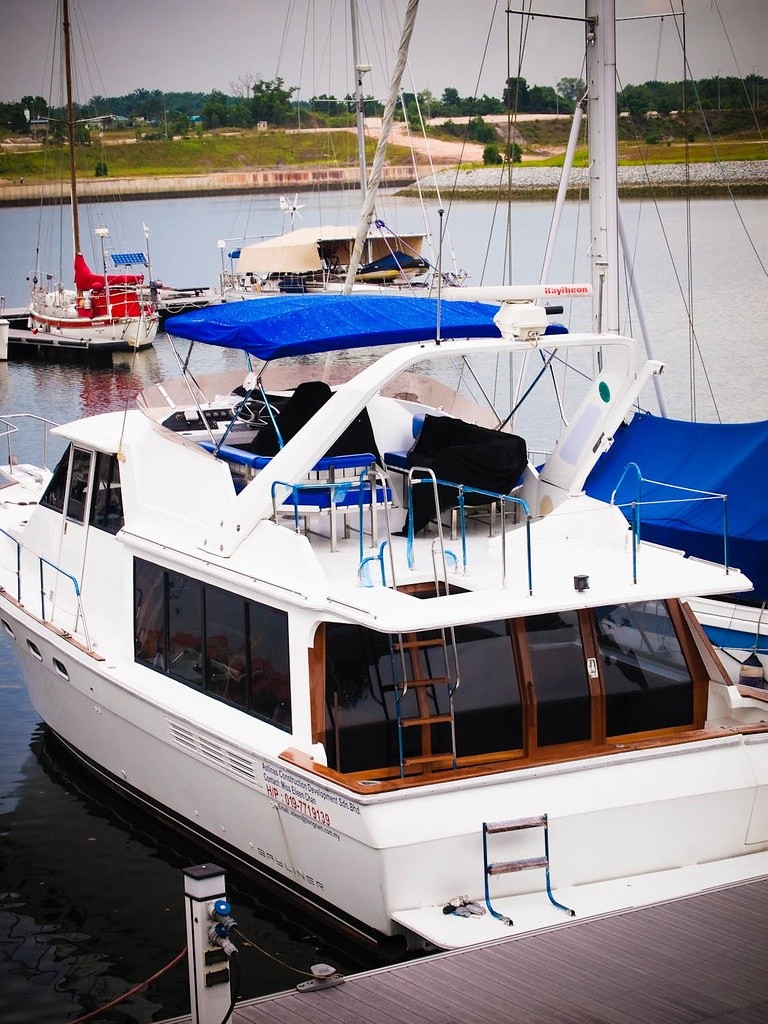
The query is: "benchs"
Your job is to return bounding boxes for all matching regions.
[384,412,525,541]
[232,461,399,551]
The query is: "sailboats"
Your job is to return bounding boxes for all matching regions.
[1,2,768,959]
